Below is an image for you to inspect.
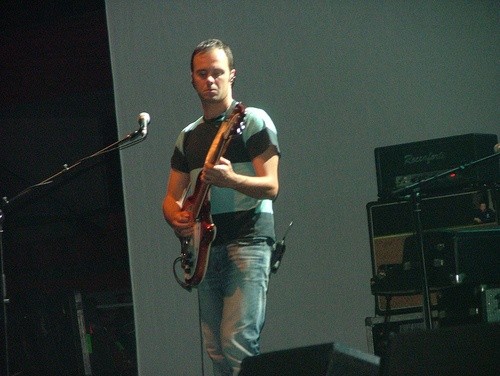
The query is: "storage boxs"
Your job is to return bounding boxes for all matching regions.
[60,291,138,376]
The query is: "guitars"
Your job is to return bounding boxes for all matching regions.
[177,101,247,290]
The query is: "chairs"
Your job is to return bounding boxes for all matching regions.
[369,234,457,376]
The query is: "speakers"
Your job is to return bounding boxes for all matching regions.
[237,341,381,376]
[381,322,500,376]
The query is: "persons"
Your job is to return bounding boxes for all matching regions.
[160,38,282,376]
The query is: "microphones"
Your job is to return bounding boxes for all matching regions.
[138,112,150,137]
